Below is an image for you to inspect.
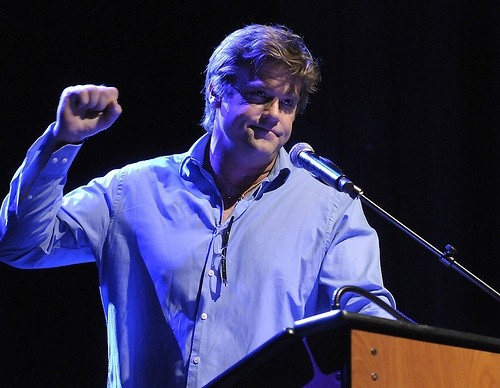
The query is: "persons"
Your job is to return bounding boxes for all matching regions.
[0,24,399,388]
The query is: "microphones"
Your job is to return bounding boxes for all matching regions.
[289,142,354,193]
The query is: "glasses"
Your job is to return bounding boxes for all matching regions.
[212,215,234,287]
